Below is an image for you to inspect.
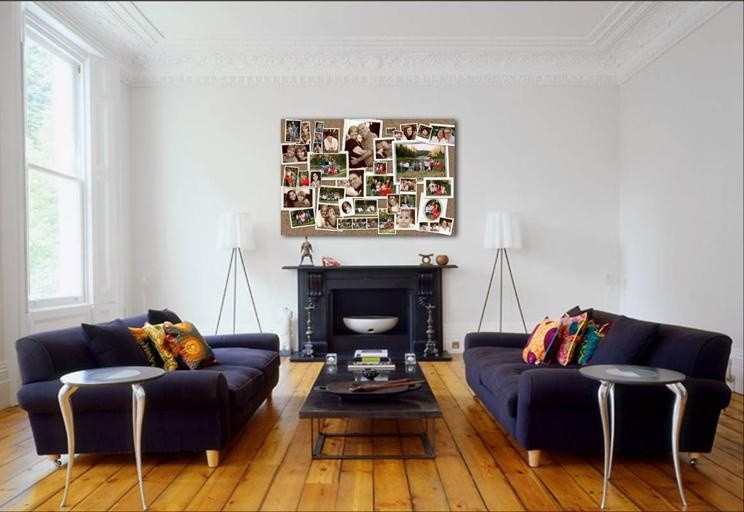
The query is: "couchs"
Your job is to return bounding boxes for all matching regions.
[463,306,733,466]
[14,308,281,468]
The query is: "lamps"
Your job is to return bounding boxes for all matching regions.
[215,209,264,335]
[476,212,529,333]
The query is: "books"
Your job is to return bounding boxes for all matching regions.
[347,347,397,371]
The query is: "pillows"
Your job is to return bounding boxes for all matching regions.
[521,316,567,366]
[145,322,184,370]
[127,324,164,370]
[163,321,219,370]
[557,312,587,366]
[586,315,659,365]
[562,306,594,320]
[147,309,182,324]
[575,319,611,364]
[80,319,149,366]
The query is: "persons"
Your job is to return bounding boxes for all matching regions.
[280,121,456,235]
[300,235,315,265]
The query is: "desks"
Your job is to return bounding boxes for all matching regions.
[578,365,689,511]
[57,365,167,512]
